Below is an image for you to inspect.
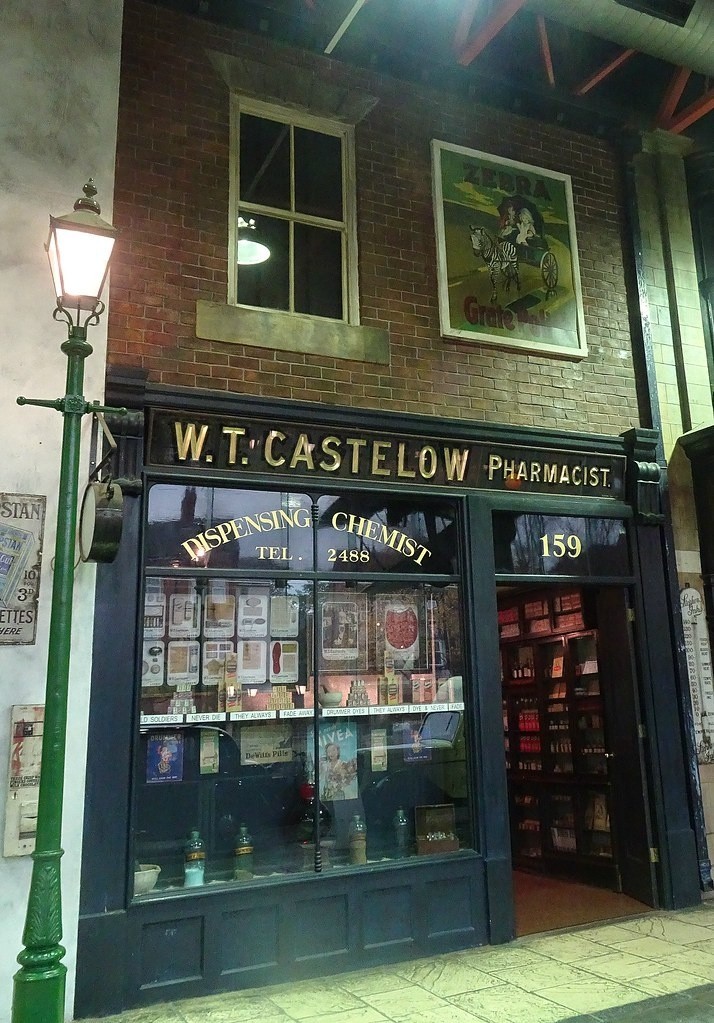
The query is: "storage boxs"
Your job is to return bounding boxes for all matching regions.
[550,823,576,854]
[502,625,520,638]
[413,804,459,856]
[499,608,519,623]
[265,686,294,710]
[524,601,548,617]
[216,678,242,712]
[528,619,550,632]
[385,651,395,674]
[410,674,436,703]
[378,674,403,706]
[555,613,583,628]
[166,684,196,714]
[553,594,580,611]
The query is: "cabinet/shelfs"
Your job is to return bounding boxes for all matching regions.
[499,629,607,774]
[508,776,623,892]
[497,586,585,642]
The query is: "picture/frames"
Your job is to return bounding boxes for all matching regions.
[429,137,588,361]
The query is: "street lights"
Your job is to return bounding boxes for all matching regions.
[11,178,128,1023]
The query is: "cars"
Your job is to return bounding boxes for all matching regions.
[328,676,474,831]
[137,727,332,865]
